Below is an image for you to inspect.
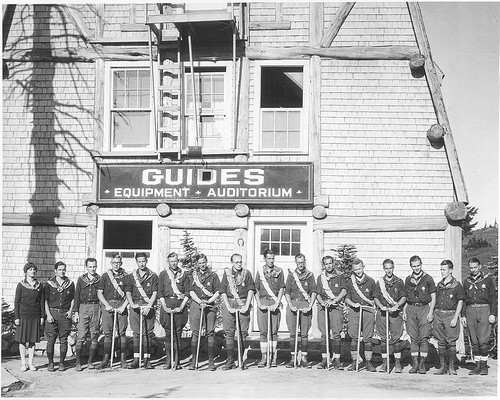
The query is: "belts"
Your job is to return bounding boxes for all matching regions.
[437,309,456,312]
[170,296,179,299]
[49,308,69,312]
[228,298,247,301]
[108,300,125,303]
[80,301,97,304]
[291,297,306,301]
[408,303,428,306]
[469,303,490,307]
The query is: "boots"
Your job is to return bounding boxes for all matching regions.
[239,348,249,369]
[120,352,128,369]
[222,348,235,370]
[98,353,110,370]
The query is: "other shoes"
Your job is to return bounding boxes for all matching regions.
[59,363,65,371]
[128,359,139,368]
[189,359,198,370]
[144,359,153,369]
[48,363,54,372]
[176,361,182,369]
[258,358,268,366]
[21,365,26,371]
[75,362,82,371]
[28,364,36,370]
[164,359,170,369]
[208,361,216,371]
[270,355,278,366]
[284,352,488,375]
[88,361,95,368]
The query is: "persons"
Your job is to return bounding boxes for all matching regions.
[74,258,101,371]
[219,254,255,370]
[253,250,286,367]
[433,260,466,375]
[96,254,128,370]
[188,253,220,371]
[463,258,497,375]
[157,252,190,370]
[343,259,375,372]
[284,253,317,368]
[405,256,436,374]
[125,253,159,369]
[372,259,408,373]
[14,262,45,372]
[316,256,348,370]
[43,261,75,372]
